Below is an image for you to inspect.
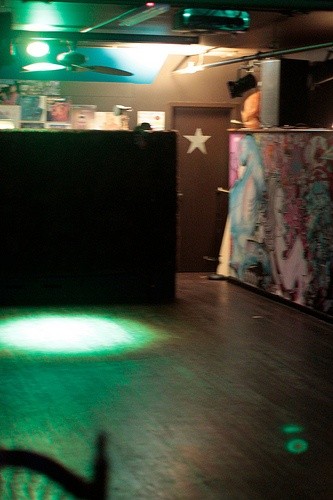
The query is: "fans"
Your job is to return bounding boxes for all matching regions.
[18,41,136,77]
